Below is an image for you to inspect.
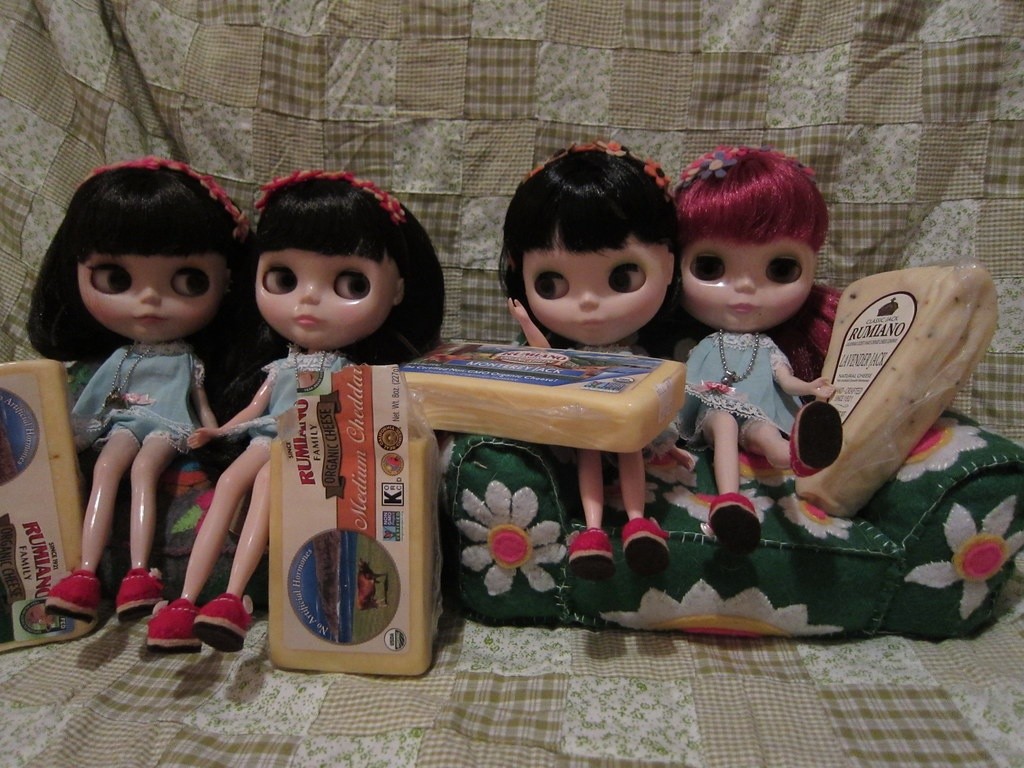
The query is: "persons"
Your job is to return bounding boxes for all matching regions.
[495,139,844,578]
[28,156,446,650]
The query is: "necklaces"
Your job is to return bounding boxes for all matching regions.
[112,345,149,389]
[716,327,759,386]
[293,349,326,379]
